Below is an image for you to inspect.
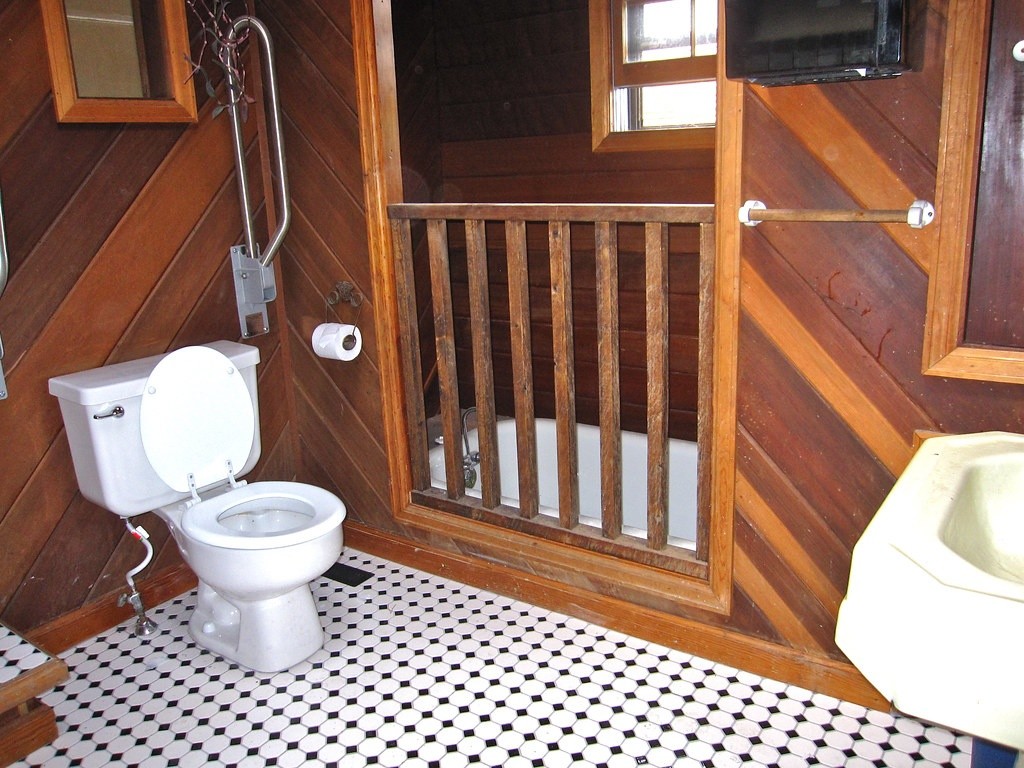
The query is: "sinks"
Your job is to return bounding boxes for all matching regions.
[836,429,1024,750]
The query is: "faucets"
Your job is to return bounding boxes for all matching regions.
[461,405,478,456]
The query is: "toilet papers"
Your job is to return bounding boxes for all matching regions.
[311,322,362,362]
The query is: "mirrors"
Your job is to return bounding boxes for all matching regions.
[39,0,199,124]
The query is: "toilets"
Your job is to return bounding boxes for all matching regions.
[48,339,348,671]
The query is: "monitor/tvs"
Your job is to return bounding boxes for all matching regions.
[726,0,909,82]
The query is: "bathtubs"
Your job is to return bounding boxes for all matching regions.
[427,416,697,553]
